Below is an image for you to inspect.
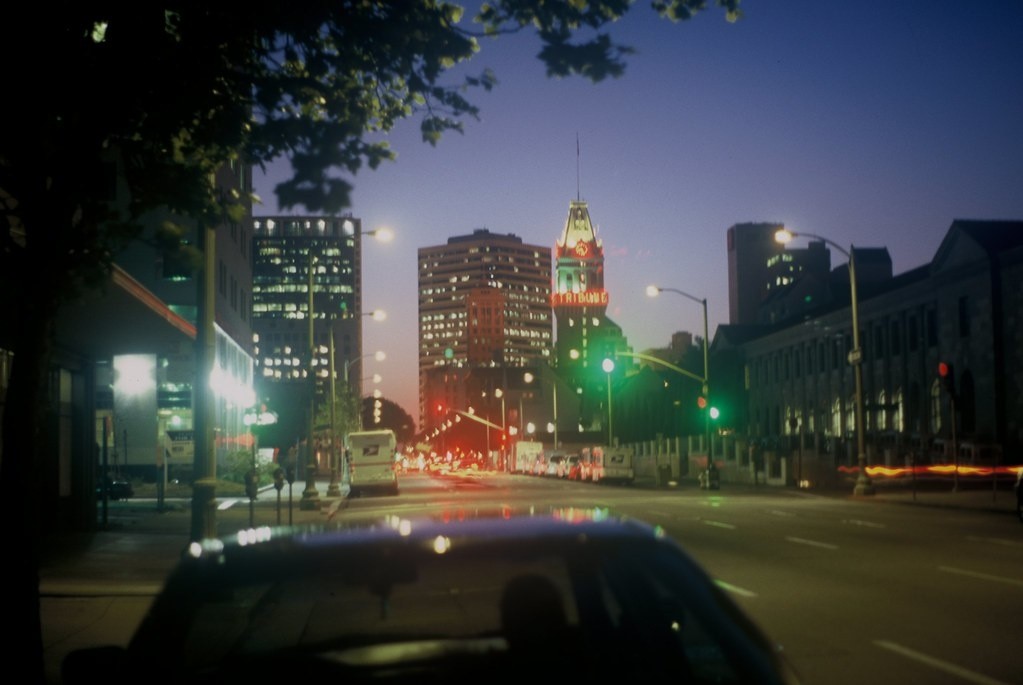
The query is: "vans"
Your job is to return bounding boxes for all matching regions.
[347,429,399,498]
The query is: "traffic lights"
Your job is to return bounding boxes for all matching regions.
[937,360,954,396]
[603,339,618,373]
[708,390,720,421]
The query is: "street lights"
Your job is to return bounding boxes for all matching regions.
[775,229,874,498]
[645,285,723,492]
[416,347,615,449]
[296,223,392,509]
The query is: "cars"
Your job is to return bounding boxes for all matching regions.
[55,511,800,685]
[401,445,638,483]
